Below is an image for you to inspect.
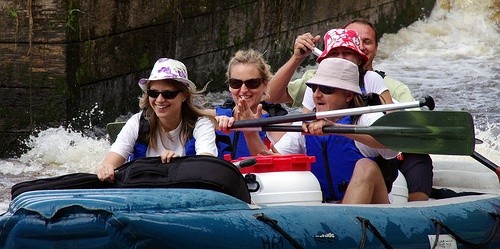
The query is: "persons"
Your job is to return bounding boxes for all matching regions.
[95,58,218,183]
[204,19,434,205]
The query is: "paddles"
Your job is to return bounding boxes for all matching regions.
[229,110,475,155]
[300,40,500,185]
[105,96,436,145]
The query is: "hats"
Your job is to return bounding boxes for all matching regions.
[137,57,197,96]
[304,57,362,95]
[316,29,368,68]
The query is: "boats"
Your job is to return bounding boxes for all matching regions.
[0,128,500,249]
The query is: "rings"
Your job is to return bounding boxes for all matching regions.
[105,172,108,175]
[314,125,318,126]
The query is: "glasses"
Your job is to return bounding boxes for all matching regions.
[312,84,335,95]
[147,88,182,99]
[229,77,264,90]
